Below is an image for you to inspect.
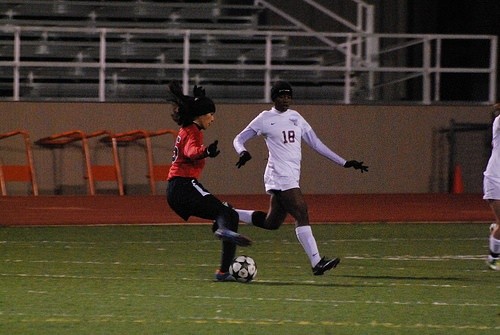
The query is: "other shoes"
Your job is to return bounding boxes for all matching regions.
[214,228,252,248]
[311,256,340,276]
[216,272,238,282]
[487,253,499,271]
[210,201,233,233]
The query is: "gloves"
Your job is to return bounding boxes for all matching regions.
[236,151,252,169]
[345,160,369,173]
[203,140,220,158]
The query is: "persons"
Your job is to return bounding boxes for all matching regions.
[209,81,370,279]
[480,112,500,271]
[166,92,253,282]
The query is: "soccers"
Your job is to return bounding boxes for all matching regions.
[229,255,258,283]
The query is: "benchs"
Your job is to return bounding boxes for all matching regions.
[0,0,369,102]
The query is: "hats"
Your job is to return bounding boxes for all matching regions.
[271,81,293,102]
